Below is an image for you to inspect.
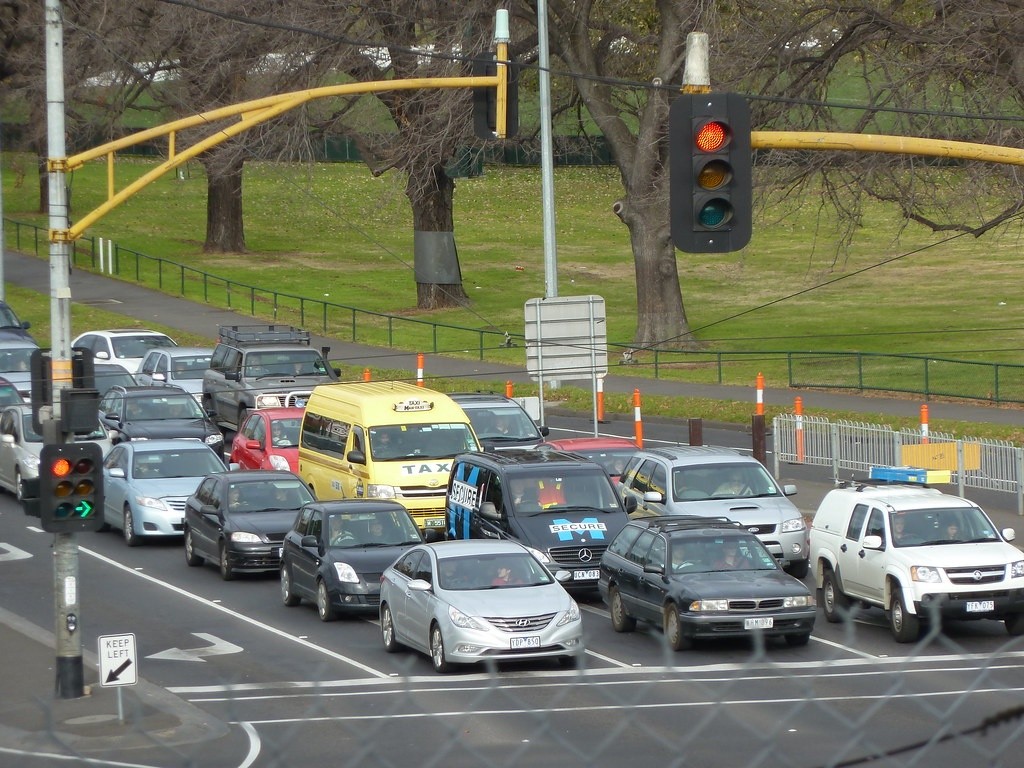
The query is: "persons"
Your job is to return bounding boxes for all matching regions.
[369,517,389,541]
[510,478,534,506]
[715,545,751,569]
[271,423,292,446]
[946,518,962,540]
[891,515,905,539]
[671,544,693,570]
[710,470,754,495]
[329,518,355,546]
[229,486,248,509]
[292,360,304,372]
[489,417,511,434]
[490,563,522,586]
[614,460,625,473]
[129,401,140,417]
[273,488,289,502]
[380,432,392,448]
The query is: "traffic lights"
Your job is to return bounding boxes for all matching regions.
[670,89,752,255]
[41,443,101,529]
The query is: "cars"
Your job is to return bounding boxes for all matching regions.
[70,330,182,379]
[228,406,315,478]
[100,439,232,547]
[1,300,112,503]
[537,435,640,495]
[598,519,819,649]
[136,347,225,418]
[183,471,321,581]
[280,500,438,620]
[93,363,140,400]
[378,539,586,672]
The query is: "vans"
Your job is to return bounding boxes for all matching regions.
[445,447,640,603]
[299,379,482,537]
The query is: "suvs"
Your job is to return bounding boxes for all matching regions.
[201,338,342,437]
[439,392,550,453]
[98,383,226,460]
[809,468,1024,644]
[617,445,811,580]
